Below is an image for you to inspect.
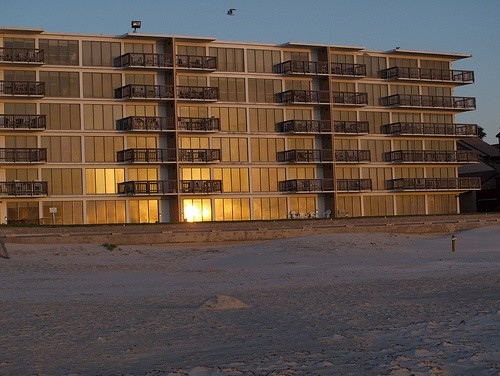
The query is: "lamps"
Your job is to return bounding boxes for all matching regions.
[131,20,141,33]
